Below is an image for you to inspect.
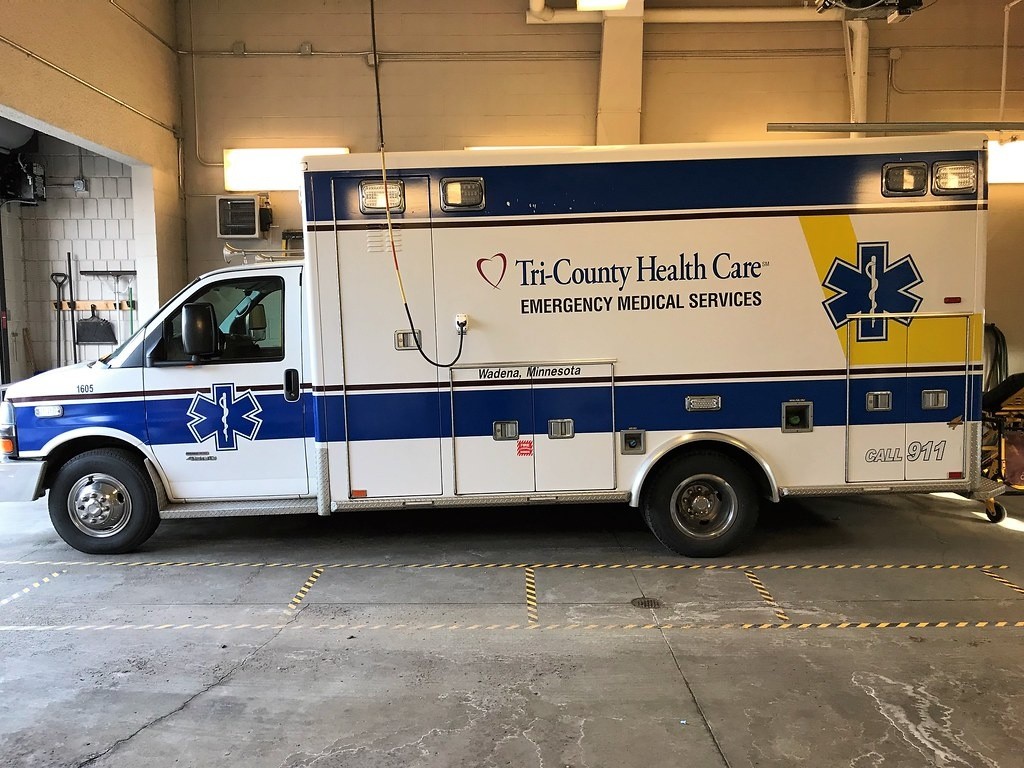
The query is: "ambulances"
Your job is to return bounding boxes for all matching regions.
[0,132,990,555]
